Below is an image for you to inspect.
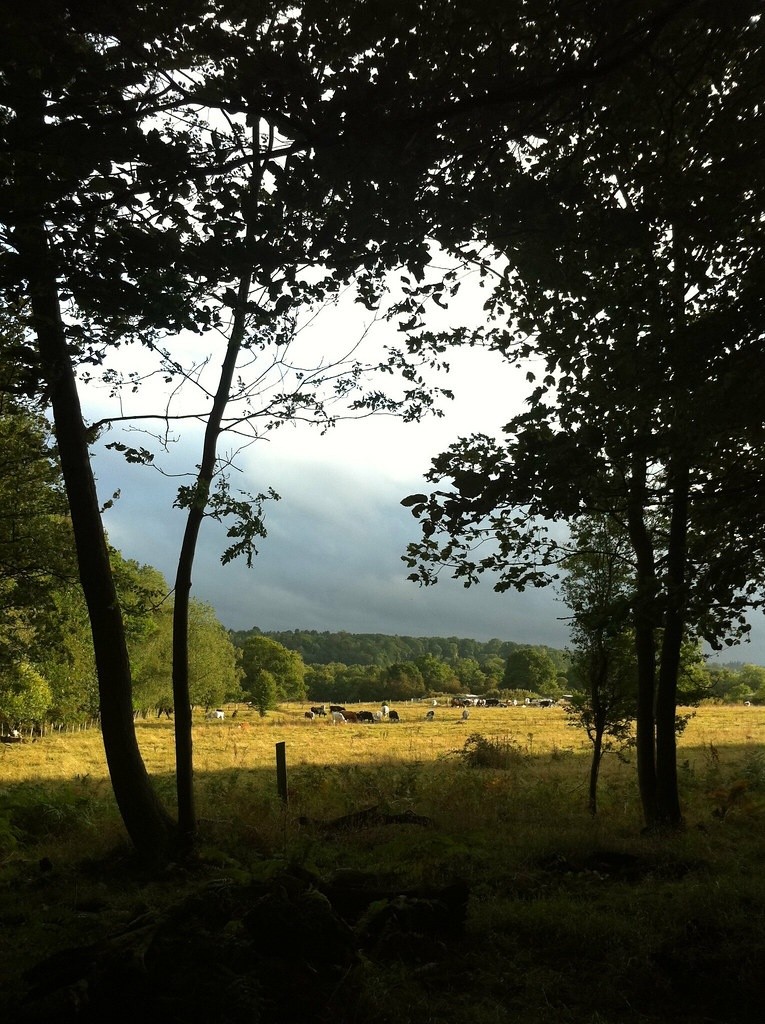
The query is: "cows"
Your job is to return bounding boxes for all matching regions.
[304,705,399,725]
[209,709,238,720]
[462,710,469,720]
[432,700,441,707]
[426,711,434,721]
[0,729,29,745]
[450,697,556,710]
